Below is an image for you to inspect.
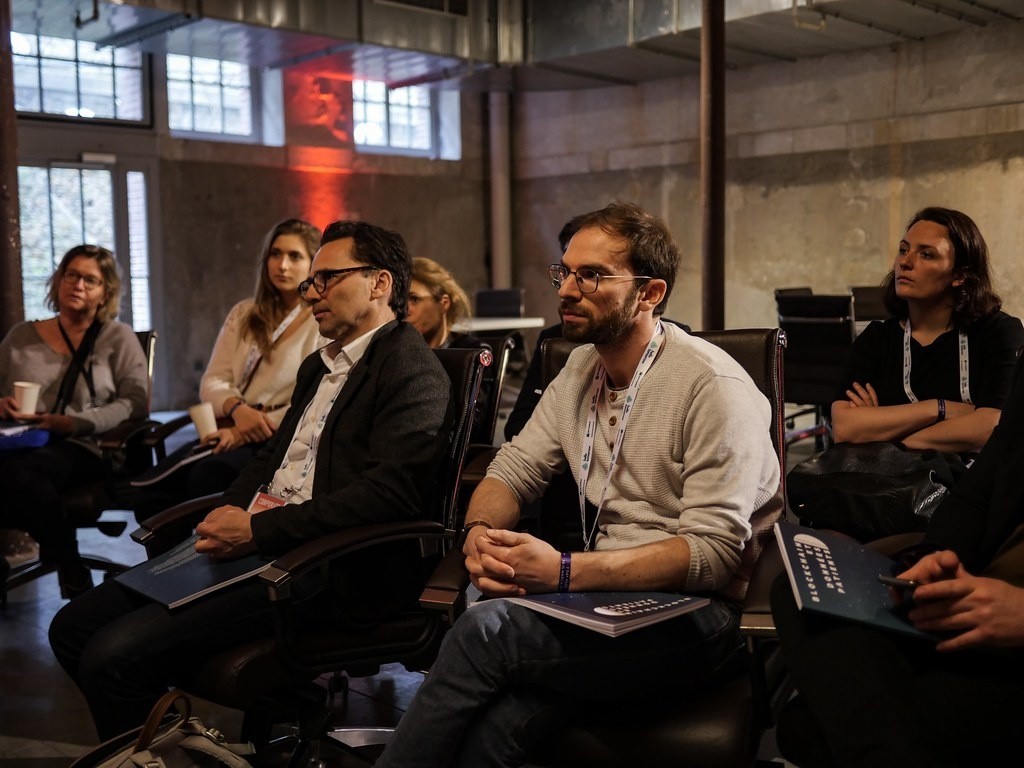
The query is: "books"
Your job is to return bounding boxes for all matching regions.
[114,534,277,609]
[774,523,943,640]
[470,591,711,637]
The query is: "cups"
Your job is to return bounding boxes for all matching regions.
[13,381,40,424]
[189,400,219,442]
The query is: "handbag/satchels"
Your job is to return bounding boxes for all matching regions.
[68,691,256,768]
[787,435,954,543]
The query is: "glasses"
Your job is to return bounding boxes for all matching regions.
[296,265,382,298]
[546,264,655,293]
[62,269,106,290]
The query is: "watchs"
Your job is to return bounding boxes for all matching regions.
[454,521,493,558]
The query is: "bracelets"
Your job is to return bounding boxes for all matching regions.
[936,399,946,422]
[558,552,571,592]
[228,401,244,417]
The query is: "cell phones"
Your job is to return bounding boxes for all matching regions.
[878,574,926,593]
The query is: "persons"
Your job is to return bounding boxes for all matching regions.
[0,244,148,599]
[787,207,1024,538]
[375,203,783,768]
[770,353,1024,768]
[405,258,494,438]
[128,218,335,553]
[47,220,450,768]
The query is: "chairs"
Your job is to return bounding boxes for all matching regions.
[0,287,1024,768]
